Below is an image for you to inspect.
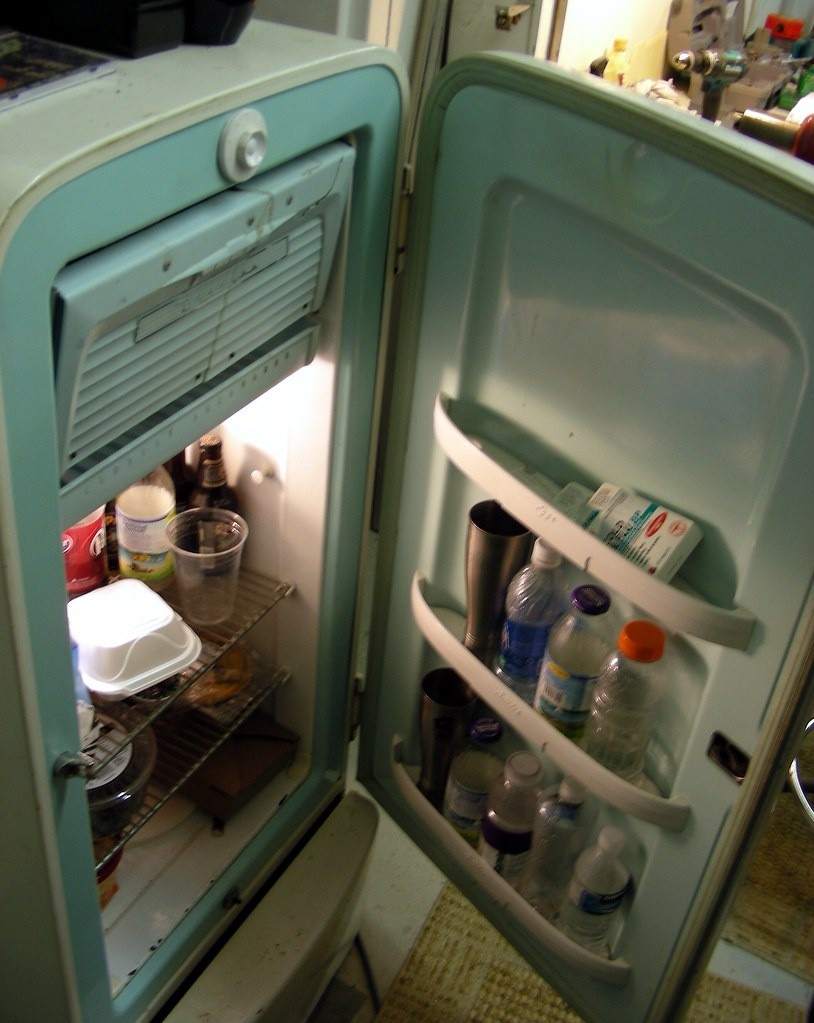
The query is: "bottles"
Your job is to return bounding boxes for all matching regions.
[477,750,547,895]
[519,775,597,926]
[490,536,570,704]
[162,448,195,514]
[116,464,176,591]
[189,433,239,575]
[62,497,118,594]
[533,583,617,745]
[579,621,668,787]
[557,825,630,954]
[443,718,505,850]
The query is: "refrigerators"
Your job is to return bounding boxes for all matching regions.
[1,17,813,1023]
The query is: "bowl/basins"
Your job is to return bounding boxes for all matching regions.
[66,577,202,704]
[78,700,158,836]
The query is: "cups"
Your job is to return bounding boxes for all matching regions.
[460,499,535,671]
[414,668,484,816]
[162,507,249,627]
